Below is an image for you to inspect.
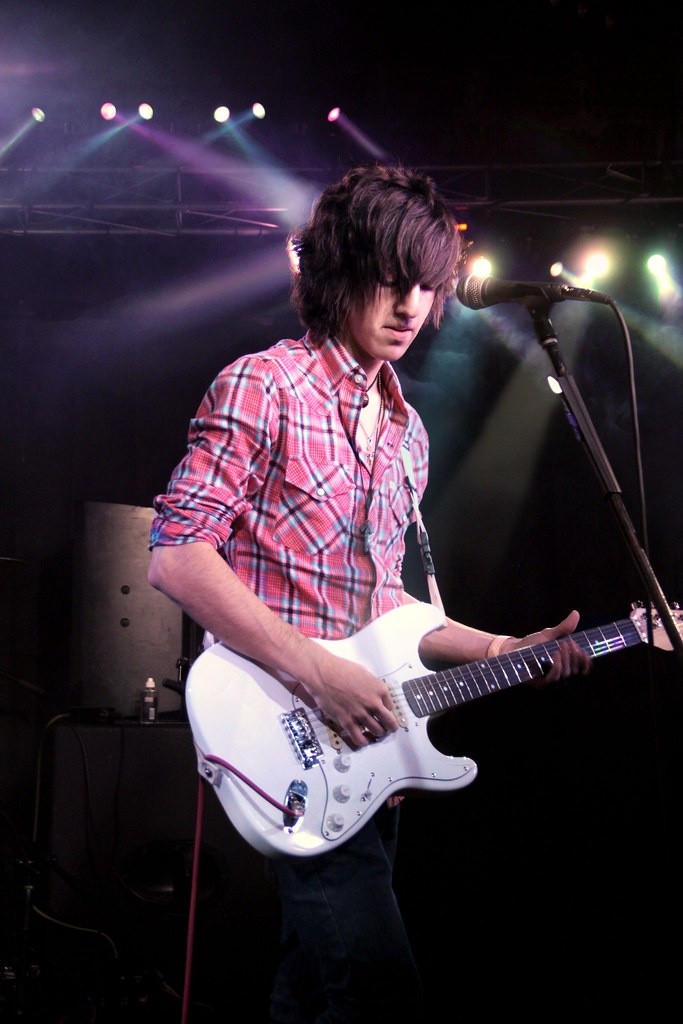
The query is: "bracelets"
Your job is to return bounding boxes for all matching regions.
[489,635,513,657]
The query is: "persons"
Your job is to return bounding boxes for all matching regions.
[148,165,590,1023]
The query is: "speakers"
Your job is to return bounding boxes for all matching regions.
[70,501,185,718]
[50,722,278,905]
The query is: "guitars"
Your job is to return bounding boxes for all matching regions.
[183,602,683,860]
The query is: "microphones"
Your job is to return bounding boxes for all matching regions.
[457,273,611,311]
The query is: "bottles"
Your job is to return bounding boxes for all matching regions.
[138,678,159,724]
